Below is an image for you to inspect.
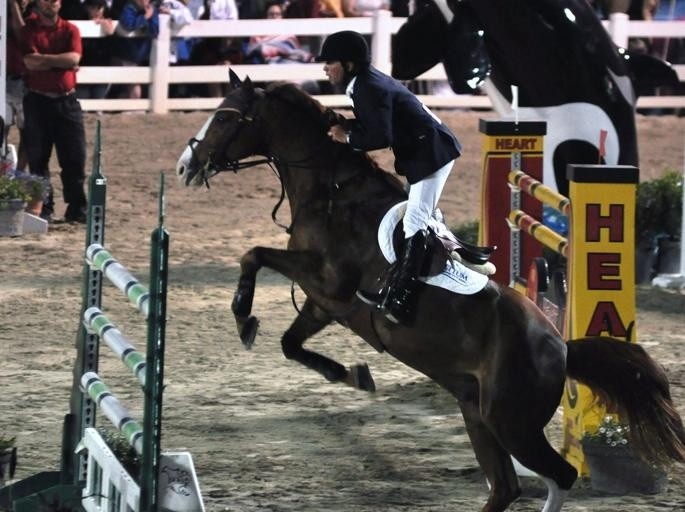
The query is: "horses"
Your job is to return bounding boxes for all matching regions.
[174,67,685,512]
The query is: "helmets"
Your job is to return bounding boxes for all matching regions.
[315,31,369,73]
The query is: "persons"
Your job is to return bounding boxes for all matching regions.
[60,0,684,115]
[313,29,462,328]
[1,1,89,226]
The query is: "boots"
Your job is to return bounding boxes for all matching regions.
[356,229,433,328]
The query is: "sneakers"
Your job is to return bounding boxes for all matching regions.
[64,209,86,223]
[41,209,53,221]
